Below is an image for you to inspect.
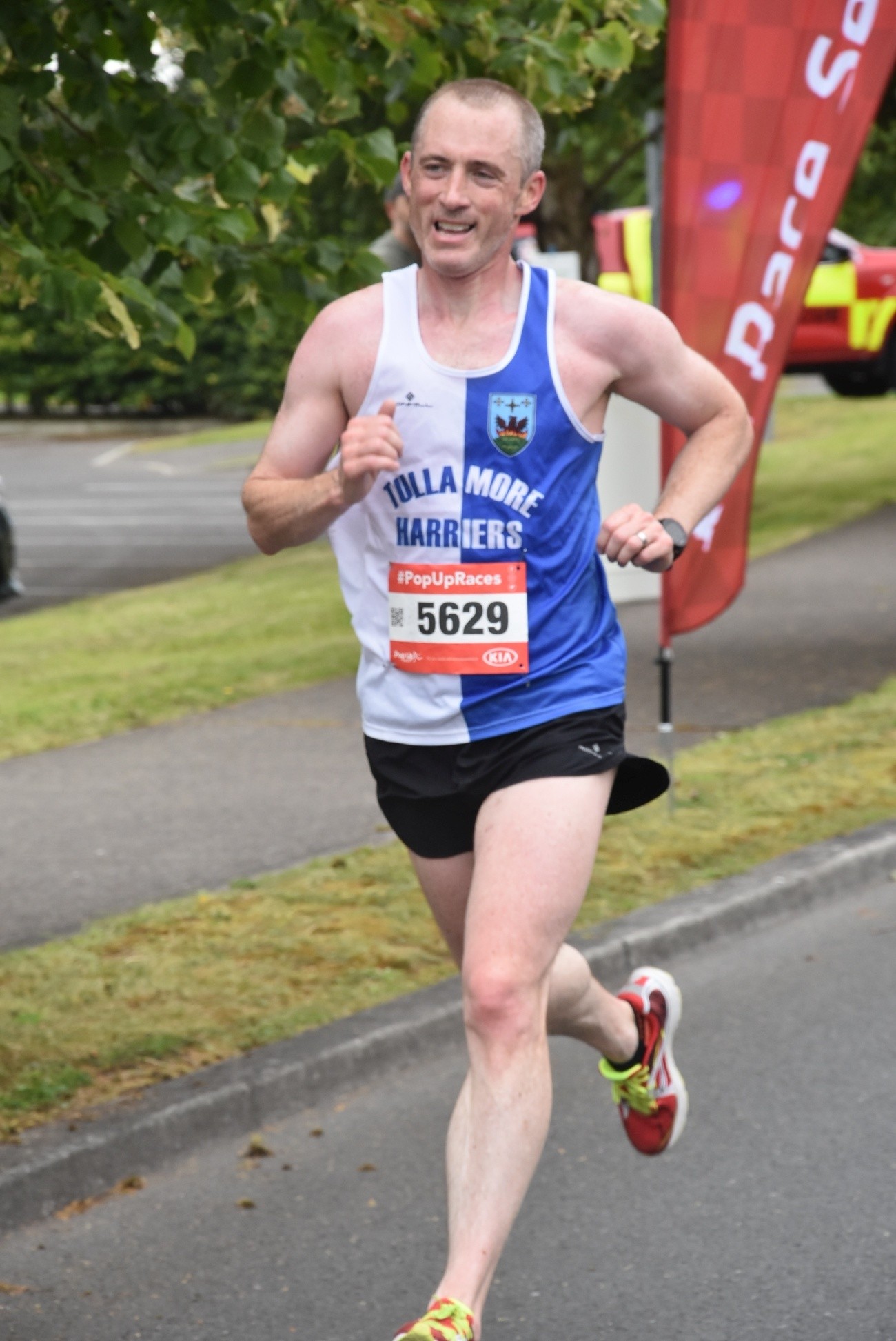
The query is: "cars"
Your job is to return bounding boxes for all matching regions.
[510,198,896,402]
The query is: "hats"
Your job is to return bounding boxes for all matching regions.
[384,172,403,205]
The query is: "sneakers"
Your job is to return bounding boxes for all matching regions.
[393,1298,475,1341]
[601,968,688,1157]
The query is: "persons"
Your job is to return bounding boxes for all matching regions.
[237,77,756,1341]
[365,170,422,272]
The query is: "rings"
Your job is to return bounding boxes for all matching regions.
[637,532,648,547]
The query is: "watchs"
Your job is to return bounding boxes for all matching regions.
[658,518,688,560]
